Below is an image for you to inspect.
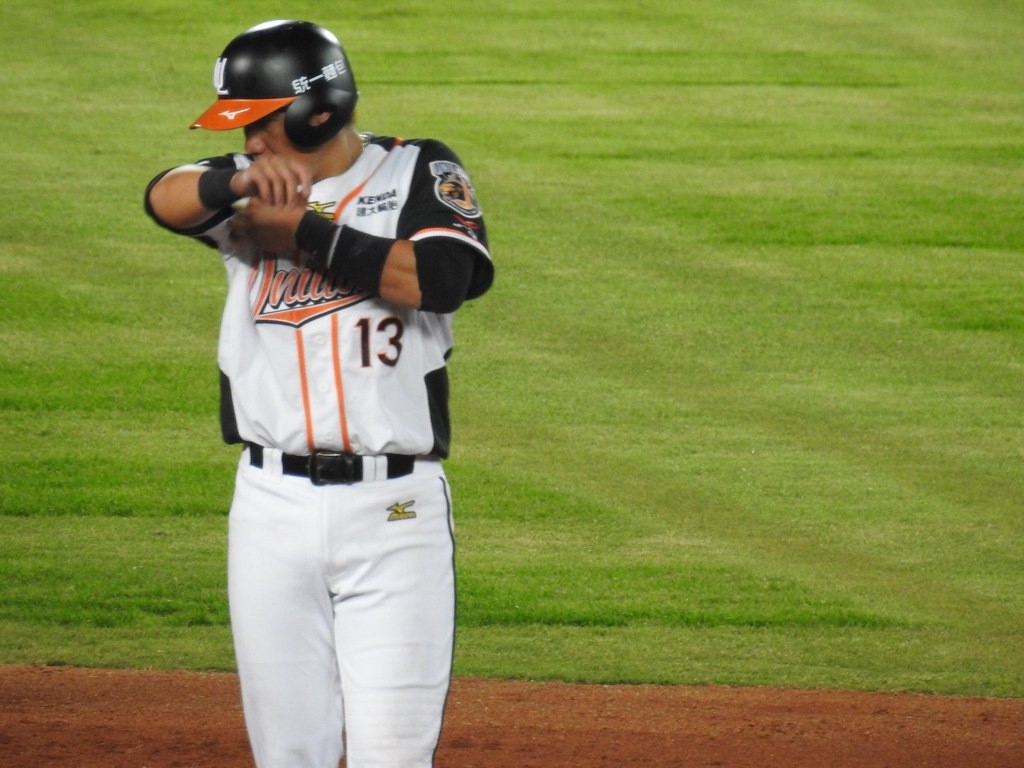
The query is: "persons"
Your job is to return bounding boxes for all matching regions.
[144,18,493,768]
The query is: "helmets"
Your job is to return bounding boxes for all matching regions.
[187,20,357,151]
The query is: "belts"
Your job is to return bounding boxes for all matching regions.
[249,443,416,486]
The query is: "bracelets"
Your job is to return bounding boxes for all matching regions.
[199,168,241,210]
[295,210,338,262]
